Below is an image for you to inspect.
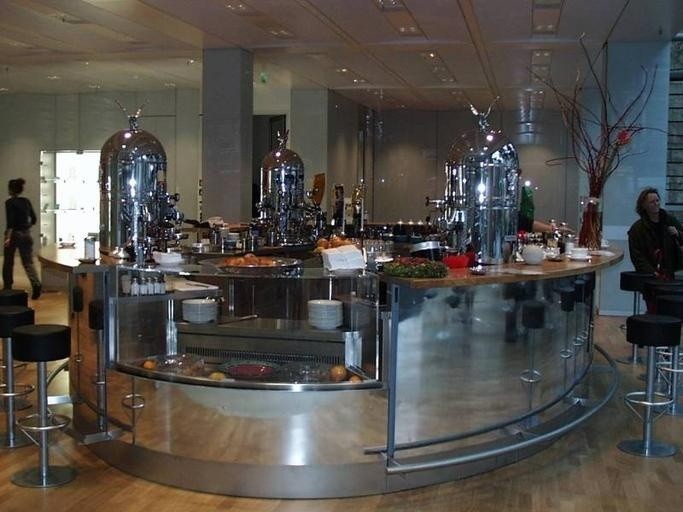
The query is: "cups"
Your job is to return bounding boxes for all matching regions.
[571,246,589,262]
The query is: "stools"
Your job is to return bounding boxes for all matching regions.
[120,374,152,467]
[1,306,38,449]
[616,271,683,458]
[562,274,596,398]
[72,286,89,406]
[1,289,36,411]
[518,302,543,425]
[11,323,78,490]
[81,301,123,444]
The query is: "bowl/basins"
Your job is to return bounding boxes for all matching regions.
[190,227,253,253]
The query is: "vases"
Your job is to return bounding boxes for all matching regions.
[579,196,603,250]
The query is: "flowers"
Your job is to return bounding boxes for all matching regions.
[527,32,669,244]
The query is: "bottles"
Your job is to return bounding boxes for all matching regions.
[84,235,96,259]
[131,273,168,296]
[509,215,592,264]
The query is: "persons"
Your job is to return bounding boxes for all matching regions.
[628,188,683,314]
[3,179,43,300]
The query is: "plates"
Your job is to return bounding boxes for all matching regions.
[77,257,96,264]
[183,297,216,325]
[306,300,342,333]
[215,358,281,380]
[59,241,75,248]
[201,255,300,276]
[154,353,210,380]
[291,366,329,384]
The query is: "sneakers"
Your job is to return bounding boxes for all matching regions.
[31,285,41,300]
